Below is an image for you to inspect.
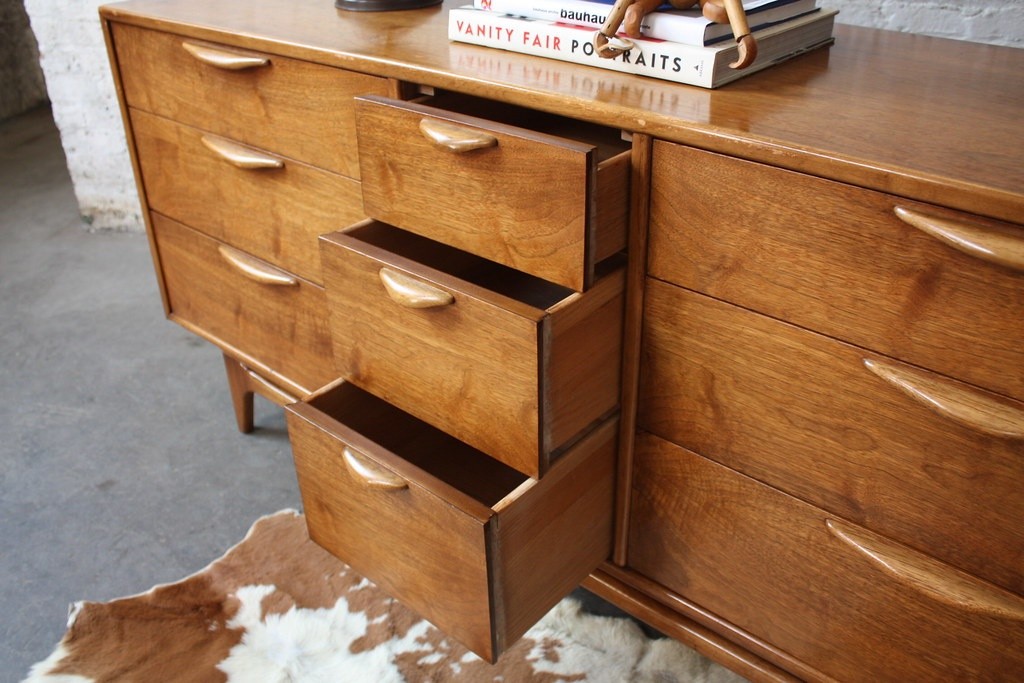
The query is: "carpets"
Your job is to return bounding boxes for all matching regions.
[14,507,753,683]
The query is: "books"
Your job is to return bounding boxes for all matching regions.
[447,1,839,93]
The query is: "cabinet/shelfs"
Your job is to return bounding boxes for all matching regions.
[92,0,1024,683]
